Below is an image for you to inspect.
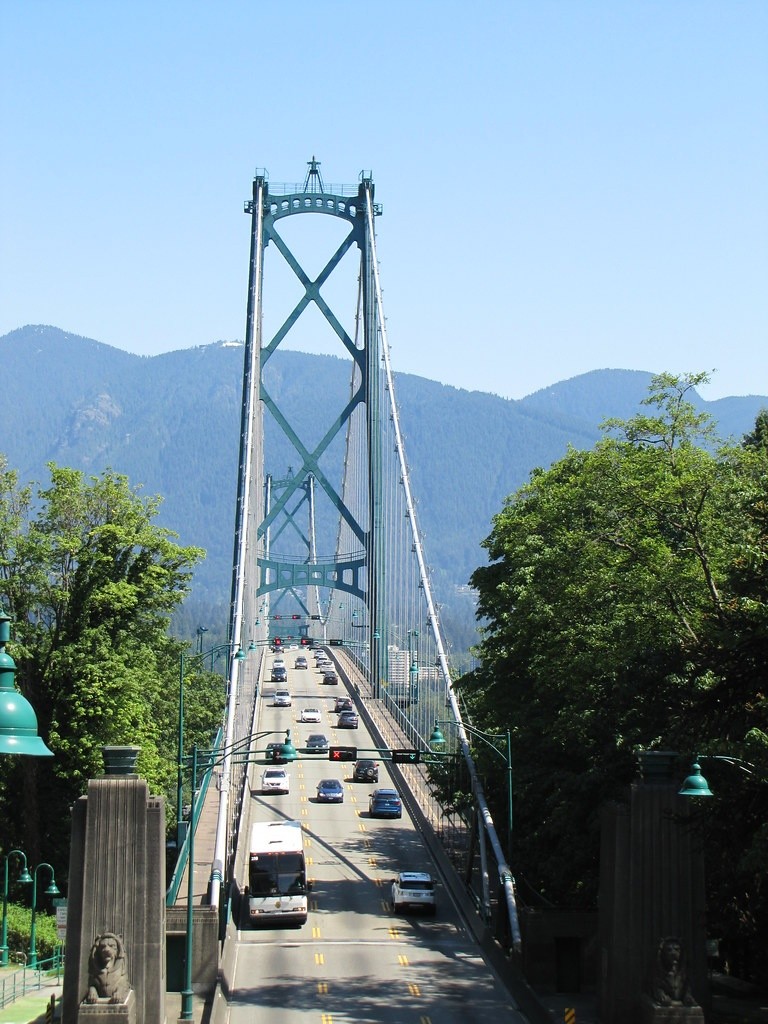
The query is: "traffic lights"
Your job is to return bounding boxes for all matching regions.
[292,615,301,619]
[275,615,281,619]
[275,638,281,645]
[329,746,357,762]
[312,616,320,620]
[393,749,419,764]
[301,638,314,645]
[329,639,342,646]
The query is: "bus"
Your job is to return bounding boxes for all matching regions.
[244,821,313,928]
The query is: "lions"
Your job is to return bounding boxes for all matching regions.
[86,932,133,1005]
[648,936,698,1007]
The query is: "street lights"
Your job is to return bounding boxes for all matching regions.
[195,627,210,674]
[0,850,34,969]
[211,638,256,674]
[180,728,299,1020]
[428,718,513,868]
[28,862,61,970]
[177,642,247,855]
[372,627,413,699]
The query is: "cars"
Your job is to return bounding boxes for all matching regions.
[301,708,322,723]
[337,710,359,729]
[305,734,329,755]
[270,642,339,685]
[265,743,287,765]
[334,697,352,713]
[273,690,291,707]
[316,778,344,804]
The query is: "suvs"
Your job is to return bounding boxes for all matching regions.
[369,789,402,819]
[391,872,437,917]
[352,759,379,783]
[260,768,290,795]
